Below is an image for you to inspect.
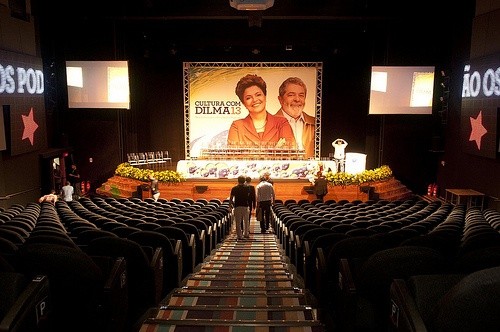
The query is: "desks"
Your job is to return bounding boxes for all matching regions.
[445,189,486,211]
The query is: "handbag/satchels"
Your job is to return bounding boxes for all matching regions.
[256,208,262,221]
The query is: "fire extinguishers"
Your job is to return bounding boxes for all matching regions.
[428,184,432,195]
[86,180,90,190]
[433,183,438,196]
[80,178,86,193]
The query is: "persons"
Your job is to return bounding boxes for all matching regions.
[60,181,74,208]
[264,171,273,185]
[39,189,57,206]
[227,73,300,160]
[256,175,275,233]
[229,175,251,240]
[314,171,328,203]
[273,76,315,159]
[151,177,160,202]
[69,164,81,196]
[241,176,256,234]
[315,166,328,175]
[332,139,348,172]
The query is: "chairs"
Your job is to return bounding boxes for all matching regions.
[127,150,172,170]
[268,199,500,332]
[0,195,236,332]
